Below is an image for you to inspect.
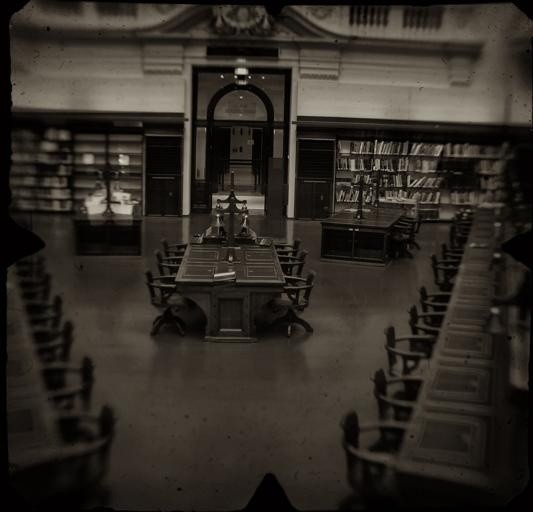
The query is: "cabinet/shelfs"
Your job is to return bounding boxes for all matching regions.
[335,136,509,221]
[9,118,143,213]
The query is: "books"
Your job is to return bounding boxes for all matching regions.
[213,271,236,282]
[7,127,144,217]
[191,235,201,244]
[258,237,274,247]
[331,138,511,219]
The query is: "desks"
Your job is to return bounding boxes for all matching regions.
[319,211,406,267]
[174,240,286,345]
[397,210,511,510]
[5,266,61,511]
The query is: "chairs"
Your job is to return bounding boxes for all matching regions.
[269,236,318,338]
[144,239,189,337]
[14,253,116,512]
[339,212,474,488]
